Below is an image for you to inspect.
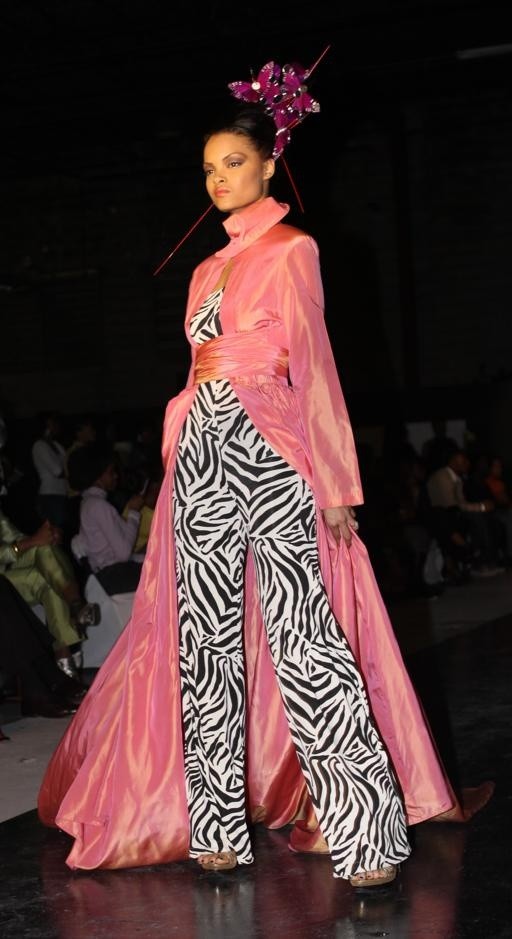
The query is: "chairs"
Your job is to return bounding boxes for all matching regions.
[82,572,135,668]
[31,603,47,626]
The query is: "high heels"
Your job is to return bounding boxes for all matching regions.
[57,657,81,683]
[67,599,101,639]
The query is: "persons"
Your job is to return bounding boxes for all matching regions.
[38,110,493,895]
[389,437,502,596]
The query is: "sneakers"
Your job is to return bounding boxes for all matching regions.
[468,560,507,579]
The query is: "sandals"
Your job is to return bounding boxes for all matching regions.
[349,861,403,888]
[198,851,239,872]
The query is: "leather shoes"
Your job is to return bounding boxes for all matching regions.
[21,693,80,720]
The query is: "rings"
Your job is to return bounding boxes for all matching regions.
[352,521,360,529]
